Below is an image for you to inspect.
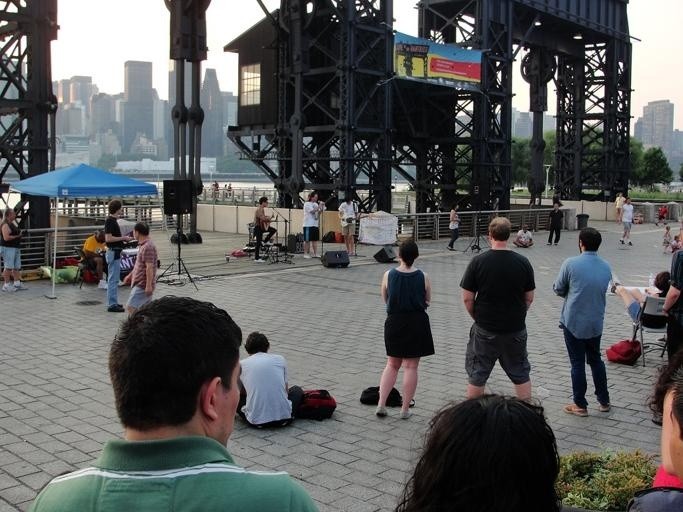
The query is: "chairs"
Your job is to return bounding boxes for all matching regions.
[71,247,110,289]
[631,297,670,367]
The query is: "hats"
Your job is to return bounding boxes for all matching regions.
[523,225,530,230]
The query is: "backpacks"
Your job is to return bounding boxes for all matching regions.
[336,232,343,243]
[300,389,336,421]
[360,386,404,406]
[322,231,336,243]
[606,339,641,366]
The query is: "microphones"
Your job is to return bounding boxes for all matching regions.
[317,200,324,204]
[353,199,358,204]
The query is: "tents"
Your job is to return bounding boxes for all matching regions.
[0,163,179,299]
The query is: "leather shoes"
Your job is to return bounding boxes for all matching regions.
[375,406,389,417]
[313,254,321,258]
[303,254,311,259]
[399,408,412,420]
[446,246,453,250]
[450,248,456,251]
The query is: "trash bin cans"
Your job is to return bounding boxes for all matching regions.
[576,214,589,230]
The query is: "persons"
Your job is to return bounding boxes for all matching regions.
[671,236,681,254]
[459,216,536,399]
[547,203,564,245]
[338,195,358,256]
[513,225,534,248]
[228,183,232,199]
[610,271,670,329]
[0,209,29,291]
[214,181,219,198]
[375,239,435,420]
[618,197,633,245]
[660,221,683,363]
[615,192,625,223]
[81,231,108,289]
[662,225,673,254]
[211,184,216,198]
[29,296,316,512]
[630,343,683,512]
[105,198,133,312]
[237,331,303,429]
[119,223,157,315]
[446,204,461,251]
[655,204,668,226]
[222,184,228,199]
[553,227,612,417]
[254,197,278,262]
[303,192,326,259]
[398,394,559,512]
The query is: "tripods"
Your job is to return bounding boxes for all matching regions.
[270,209,295,265]
[461,203,493,255]
[312,204,323,258]
[275,208,295,258]
[156,214,199,292]
[347,203,365,257]
[267,211,283,259]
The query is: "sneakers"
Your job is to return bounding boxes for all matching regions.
[2,284,18,292]
[564,403,587,417]
[255,259,266,263]
[118,303,123,307]
[598,402,611,413]
[553,242,559,245]
[547,243,552,245]
[13,282,28,290]
[346,250,354,256]
[619,240,625,245]
[627,241,632,246]
[108,305,125,312]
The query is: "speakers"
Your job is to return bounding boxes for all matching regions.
[321,251,350,268]
[373,246,397,262]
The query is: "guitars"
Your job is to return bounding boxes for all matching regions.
[340,214,370,228]
[258,212,276,231]
